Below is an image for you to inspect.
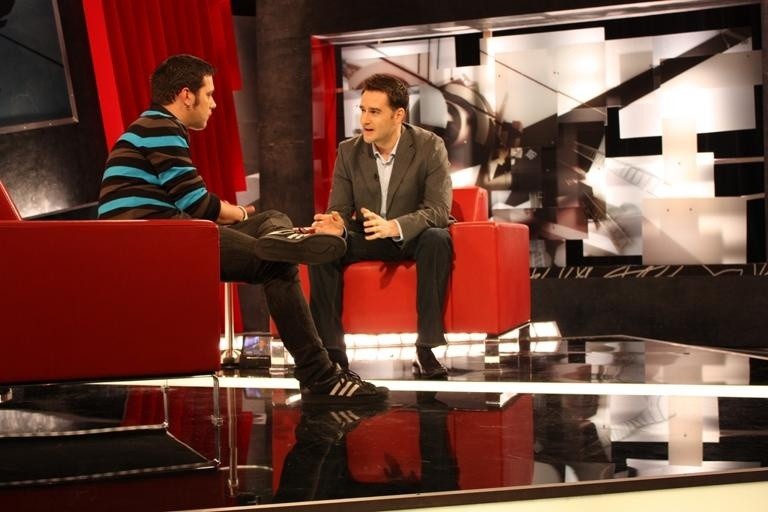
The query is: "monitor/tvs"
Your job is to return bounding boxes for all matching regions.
[1,0,79,135]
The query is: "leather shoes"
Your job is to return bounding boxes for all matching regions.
[412,350,448,380]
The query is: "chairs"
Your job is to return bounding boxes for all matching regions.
[1,181,230,403]
[269,185,541,368]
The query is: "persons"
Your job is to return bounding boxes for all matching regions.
[272,405,460,503]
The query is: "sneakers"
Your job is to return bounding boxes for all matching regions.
[253,228,348,265]
[299,363,390,407]
[294,403,390,441]
